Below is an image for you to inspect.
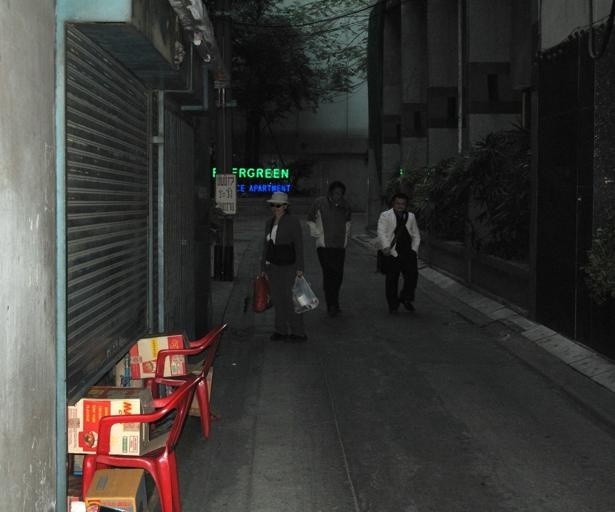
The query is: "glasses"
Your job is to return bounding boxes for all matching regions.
[270,203,282,208]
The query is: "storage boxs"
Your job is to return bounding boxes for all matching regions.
[159,364,213,409]
[130,333,187,380]
[68,387,151,456]
[115,354,143,387]
[86,469,148,512]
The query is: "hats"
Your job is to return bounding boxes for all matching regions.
[266,192,291,205]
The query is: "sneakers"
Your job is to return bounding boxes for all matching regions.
[270,333,307,342]
[327,303,340,316]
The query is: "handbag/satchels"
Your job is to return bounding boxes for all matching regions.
[377,249,389,273]
[266,247,279,264]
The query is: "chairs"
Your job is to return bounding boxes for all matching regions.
[82,372,205,512]
[144,323,228,440]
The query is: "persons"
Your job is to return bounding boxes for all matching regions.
[265,192,308,343]
[377,192,421,314]
[306,181,352,316]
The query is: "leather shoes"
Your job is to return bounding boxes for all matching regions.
[388,297,416,314]
